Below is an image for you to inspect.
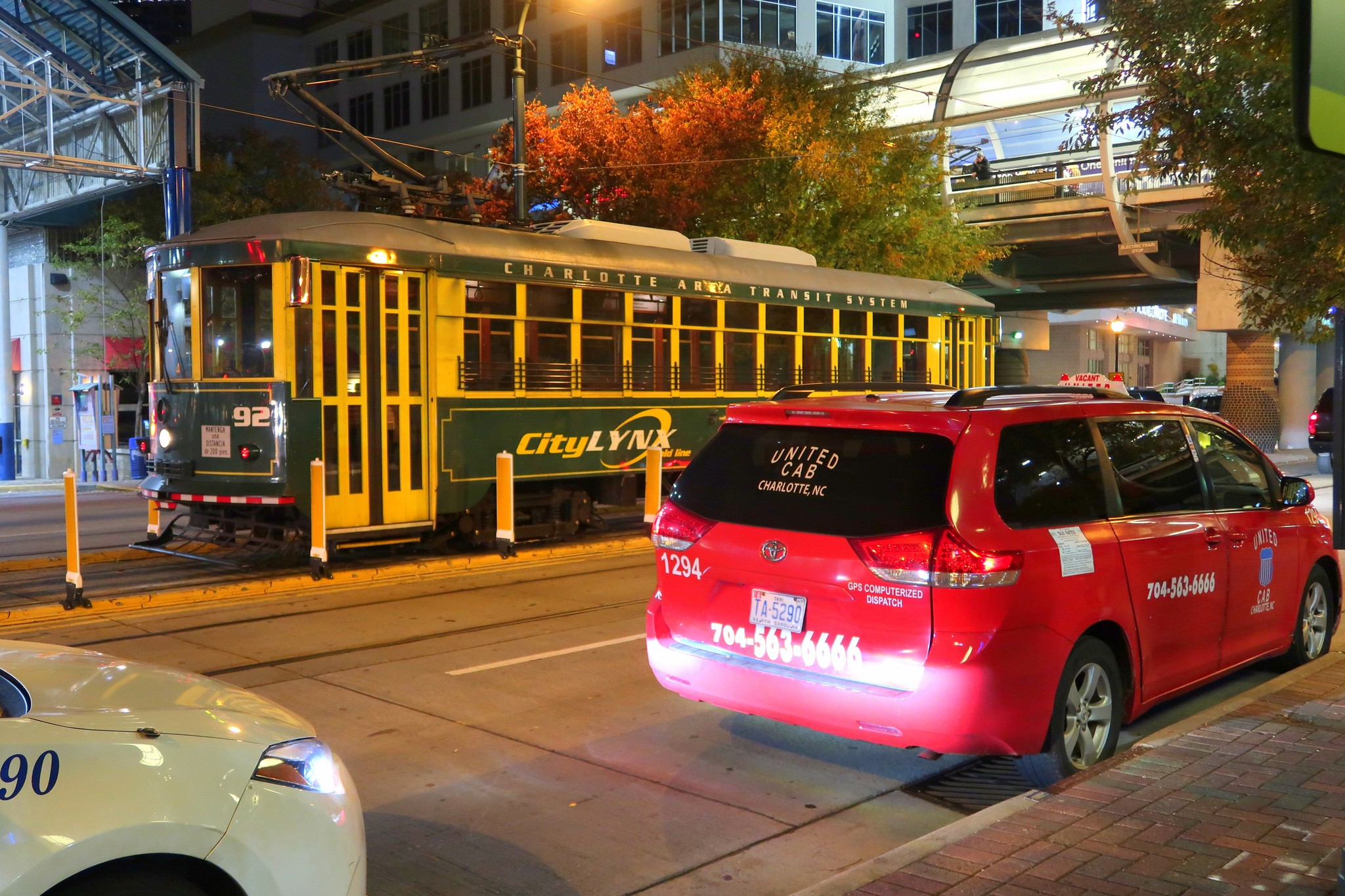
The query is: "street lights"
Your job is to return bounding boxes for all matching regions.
[1113,313,1126,378]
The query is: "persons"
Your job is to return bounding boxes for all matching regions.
[970,152,992,181]
[1065,158,1081,196]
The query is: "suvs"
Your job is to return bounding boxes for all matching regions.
[642,370,1345,794]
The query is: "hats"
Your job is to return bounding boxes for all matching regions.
[976,152,984,158]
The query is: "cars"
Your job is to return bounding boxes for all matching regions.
[1126,379,1338,460]
[0,626,367,893]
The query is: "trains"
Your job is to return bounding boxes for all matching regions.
[124,202,999,571]
